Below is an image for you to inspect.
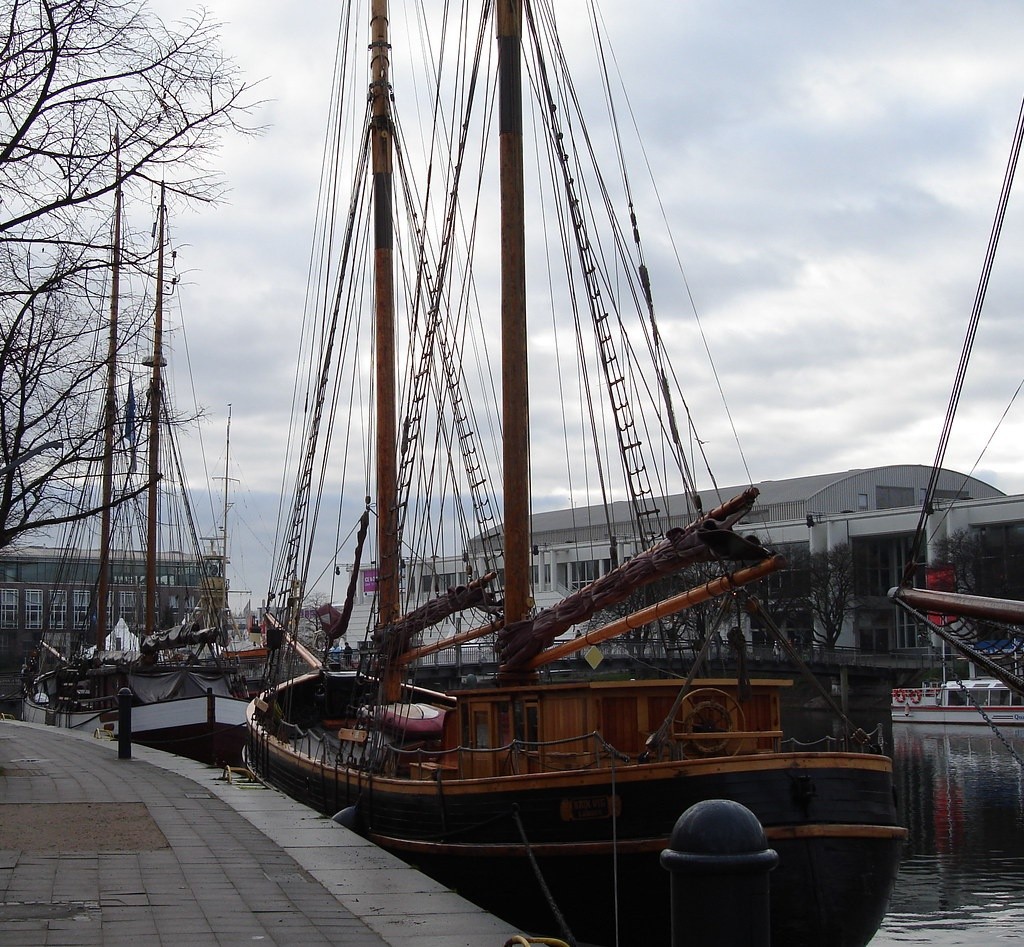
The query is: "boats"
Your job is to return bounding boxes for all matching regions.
[891,680,1024,724]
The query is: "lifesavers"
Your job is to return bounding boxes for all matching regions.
[895,688,906,704]
[910,689,921,704]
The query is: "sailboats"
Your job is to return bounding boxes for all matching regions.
[883,97,1024,769]
[21,1,908,947]
